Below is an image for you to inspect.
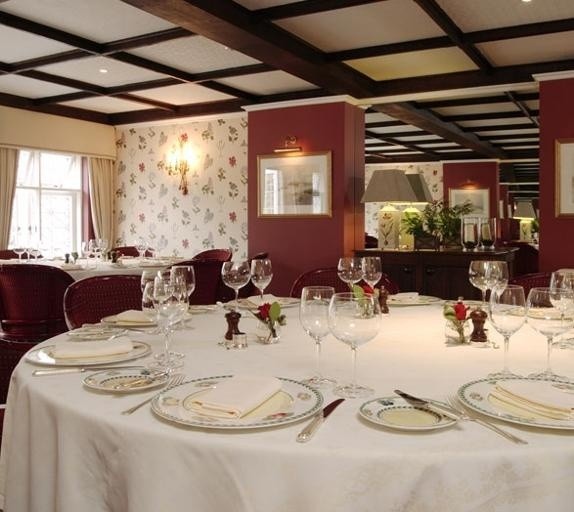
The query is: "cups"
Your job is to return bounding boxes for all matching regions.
[461,216,479,250]
[480,216,498,250]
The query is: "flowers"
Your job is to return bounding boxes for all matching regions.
[249,285,472,344]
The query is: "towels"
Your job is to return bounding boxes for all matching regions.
[490,378,574,421]
[189,372,283,419]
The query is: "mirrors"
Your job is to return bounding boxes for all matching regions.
[354,84,540,255]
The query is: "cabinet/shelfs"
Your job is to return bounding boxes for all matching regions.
[353,250,503,301]
[502,245,519,278]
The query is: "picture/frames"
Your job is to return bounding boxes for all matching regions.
[555,137,574,220]
[449,188,489,219]
[256,149,332,219]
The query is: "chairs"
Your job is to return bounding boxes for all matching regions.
[290,267,400,300]
[486,273,560,307]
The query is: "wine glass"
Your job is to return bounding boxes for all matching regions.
[250,257,274,302]
[486,283,527,378]
[329,292,382,400]
[468,257,508,342]
[143,260,195,369]
[336,256,362,317]
[550,269,574,349]
[11,237,110,266]
[300,285,339,389]
[221,259,251,315]
[360,255,381,289]
[527,287,570,379]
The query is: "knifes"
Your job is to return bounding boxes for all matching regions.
[298,396,346,443]
[392,389,464,422]
[423,397,532,448]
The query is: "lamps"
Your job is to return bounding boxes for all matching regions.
[401,173,434,250]
[360,169,418,250]
[167,143,200,194]
[273,135,302,152]
[512,202,536,240]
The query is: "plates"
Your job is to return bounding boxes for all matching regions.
[362,396,457,432]
[387,291,439,306]
[101,311,182,326]
[81,364,169,393]
[526,306,574,320]
[150,375,322,430]
[457,376,574,434]
[228,294,299,309]
[43,341,153,365]
